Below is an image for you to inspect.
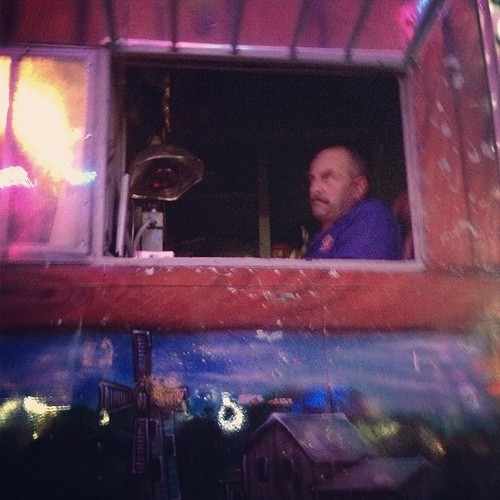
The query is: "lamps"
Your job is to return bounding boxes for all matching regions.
[126,69,206,203]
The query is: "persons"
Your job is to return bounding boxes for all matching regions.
[301,145,403,259]
